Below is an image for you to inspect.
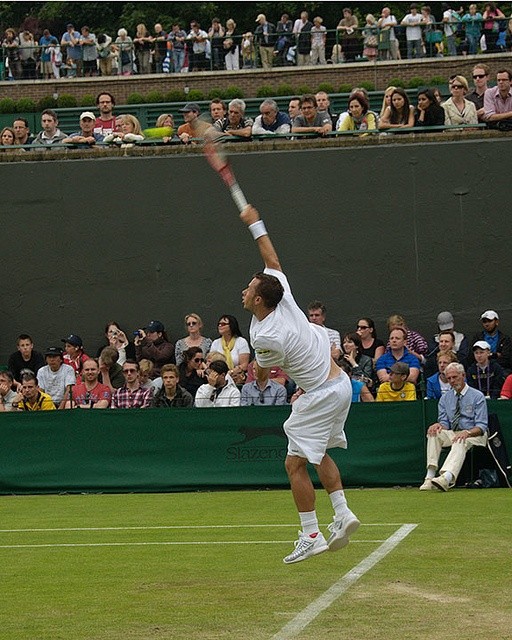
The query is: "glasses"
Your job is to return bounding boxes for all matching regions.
[193,358,204,362]
[356,325,371,330]
[451,84,465,89]
[84,368,96,371]
[217,322,228,327]
[473,74,487,78]
[186,322,196,326]
[100,101,112,105]
[122,369,135,374]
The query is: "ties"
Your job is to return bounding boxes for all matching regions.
[452,395,461,430]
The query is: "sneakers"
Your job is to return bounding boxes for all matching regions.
[327,508,360,551]
[420,480,437,491]
[432,476,451,491]
[283,530,330,564]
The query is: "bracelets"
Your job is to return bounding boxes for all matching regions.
[248,220,268,241]
[245,221,271,241]
[465,430,471,437]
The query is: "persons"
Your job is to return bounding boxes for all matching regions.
[155,114,174,145]
[0,369,19,412]
[8,334,46,393]
[379,89,415,134]
[440,75,479,132]
[30,109,69,152]
[10,374,57,411]
[133,320,175,367]
[102,114,145,149]
[110,358,153,409]
[151,364,193,408]
[484,69,512,123]
[464,63,491,130]
[239,204,360,565]
[385,314,427,365]
[375,328,420,383]
[335,360,375,403]
[94,346,126,393]
[97,322,136,366]
[338,330,377,393]
[251,100,292,141]
[356,318,386,360]
[287,378,305,403]
[71,358,112,408]
[37,345,76,410]
[418,362,488,492]
[177,346,208,400]
[61,334,91,385]
[246,357,289,386]
[337,93,377,137]
[306,300,341,360]
[177,103,211,144]
[205,98,253,142]
[137,358,160,396]
[466,340,508,399]
[175,314,212,367]
[12,117,37,151]
[208,98,228,124]
[414,88,445,133]
[288,97,303,140]
[427,311,469,358]
[379,86,398,118]
[431,88,441,103]
[424,331,468,377]
[471,310,512,369]
[1,1,512,80]
[500,374,512,400]
[292,94,333,139]
[92,92,116,145]
[0,127,27,152]
[114,114,125,132]
[336,87,380,136]
[210,314,250,384]
[241,359,288,405]
[195,361,241,407]
[62,112,106,149]
[196,351,235,387]
[375,362,417,403]
[315,91,340,138]
[426,350,467,399]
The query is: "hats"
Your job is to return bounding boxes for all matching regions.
[255,14,264,22]
[80,112,95,120]
[210,361,228,374]
[480,310,500,321]
[45,346,62,355]
[60,333,81,347]
[385,362,410,374]
[473,341,491,349]
[178,103,201,113]
[437,311,454,331]
[146,320,163,333]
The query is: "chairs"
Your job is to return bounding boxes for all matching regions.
[438,414,511,488]
